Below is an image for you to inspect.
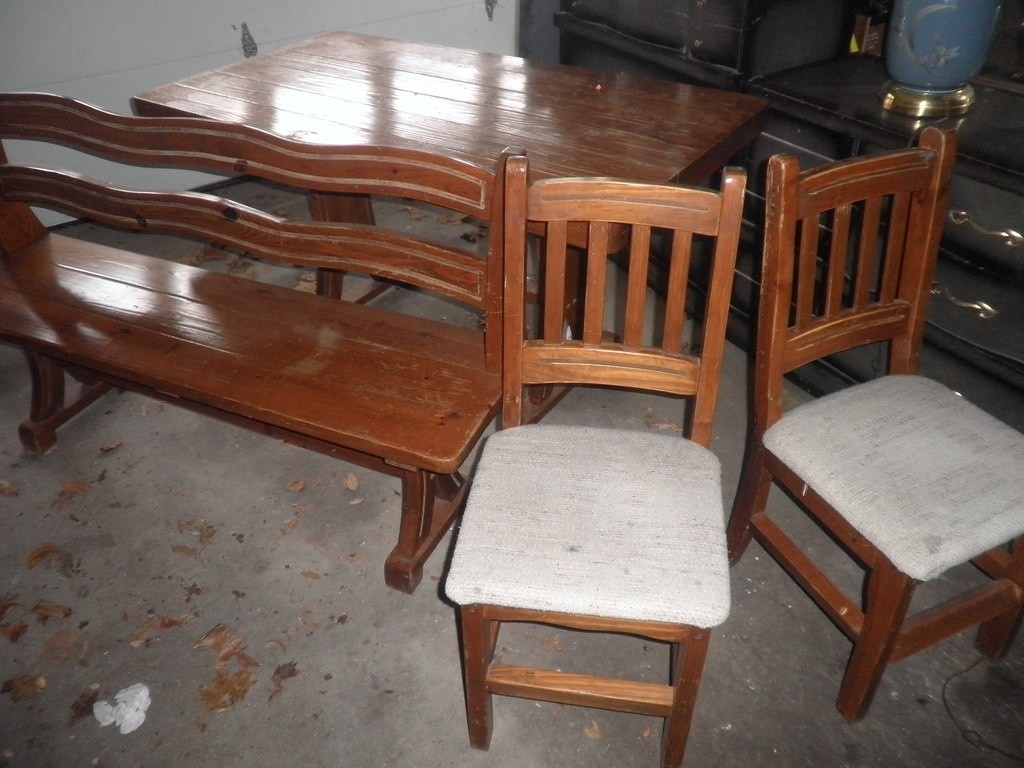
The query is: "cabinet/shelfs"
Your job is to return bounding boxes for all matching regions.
[550,2,1024,395]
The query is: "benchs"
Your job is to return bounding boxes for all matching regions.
[2,91,526,594]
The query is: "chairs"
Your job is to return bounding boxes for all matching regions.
[444,155,749,768]
[728,123,1024,726]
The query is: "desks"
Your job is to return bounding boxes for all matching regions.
[128,29,766,421]
